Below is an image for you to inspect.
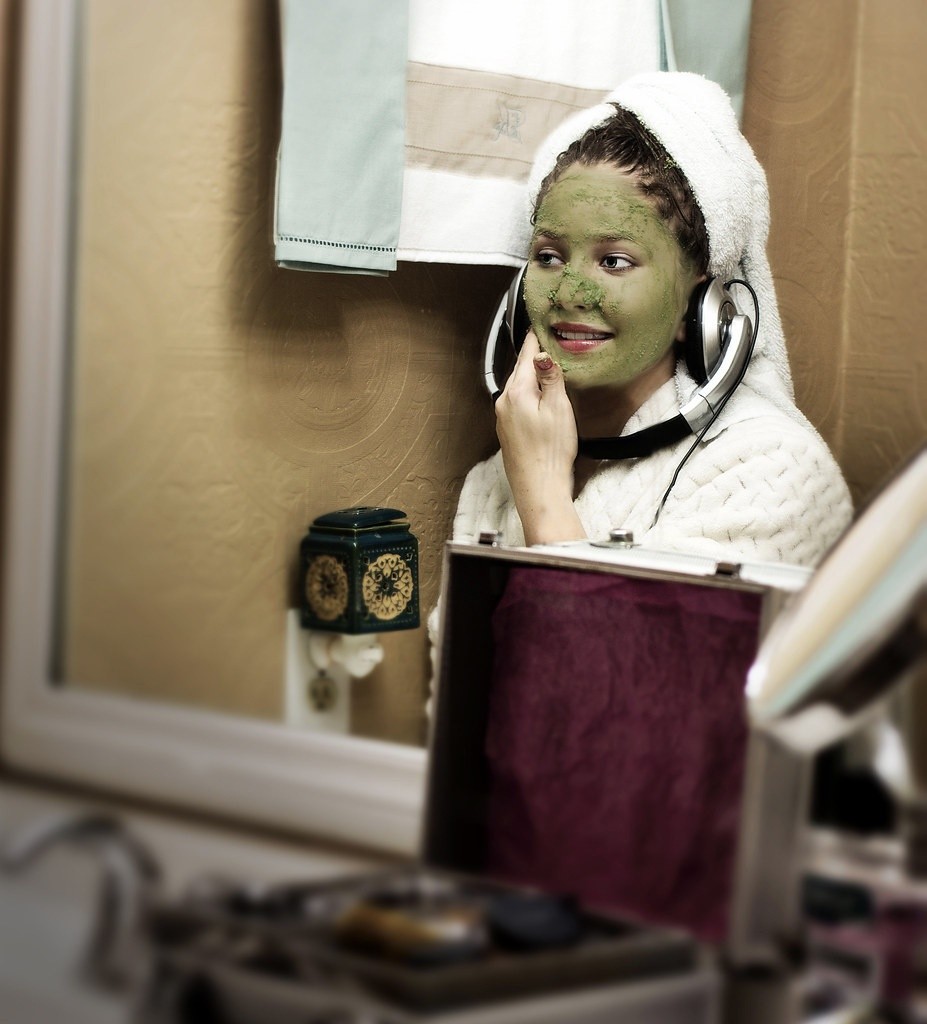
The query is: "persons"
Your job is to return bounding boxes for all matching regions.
[423,73,855,750]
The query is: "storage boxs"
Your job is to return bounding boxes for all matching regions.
[129,531,815,1024]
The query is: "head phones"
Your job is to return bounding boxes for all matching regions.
[477,260,761,459]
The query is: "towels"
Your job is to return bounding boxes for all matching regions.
[270,2,414,276]
[512,64,798,399]
[660,2,753,119]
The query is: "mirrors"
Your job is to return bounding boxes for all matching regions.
[0,0,927,868]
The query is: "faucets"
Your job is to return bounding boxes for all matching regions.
[3,807,160,987]
